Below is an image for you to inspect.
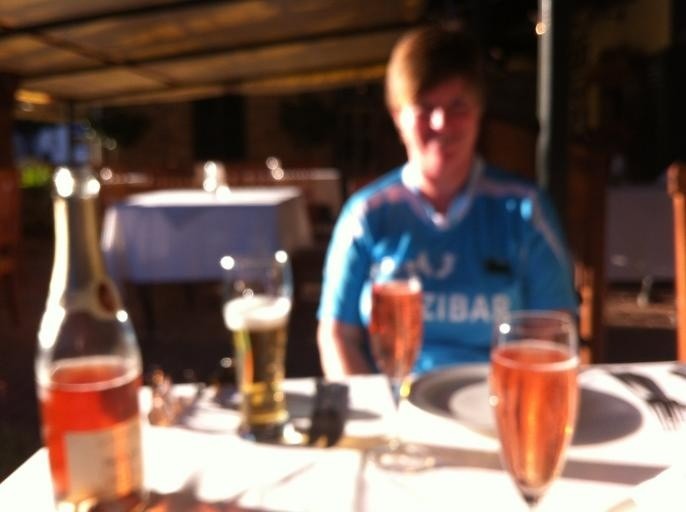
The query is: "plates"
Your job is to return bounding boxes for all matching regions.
[402,362,495,434]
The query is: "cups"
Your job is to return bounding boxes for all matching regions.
[212,251,293,431]
[488,311,580,505]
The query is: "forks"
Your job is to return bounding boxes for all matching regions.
[615,370,684,426]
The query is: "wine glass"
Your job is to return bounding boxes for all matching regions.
[362,261,438,470]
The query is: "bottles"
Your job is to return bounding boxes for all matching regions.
[31,164,149,512]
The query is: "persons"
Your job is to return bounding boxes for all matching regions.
[315,24,581,378]
